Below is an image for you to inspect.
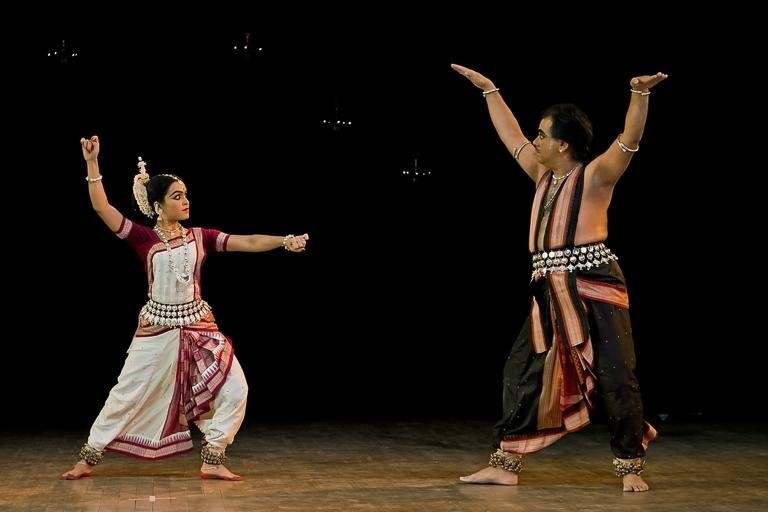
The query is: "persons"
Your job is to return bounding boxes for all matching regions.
[450,62,669,494]
[60,135,311,482]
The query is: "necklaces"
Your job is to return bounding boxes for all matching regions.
[154,223,190,287]
[156,223,181,238]
[552,167,574,187]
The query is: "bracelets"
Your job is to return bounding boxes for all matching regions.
[630,87,651,97]
[85,175,102,183]
[481,88,499,98]
[281,233,294,251]
